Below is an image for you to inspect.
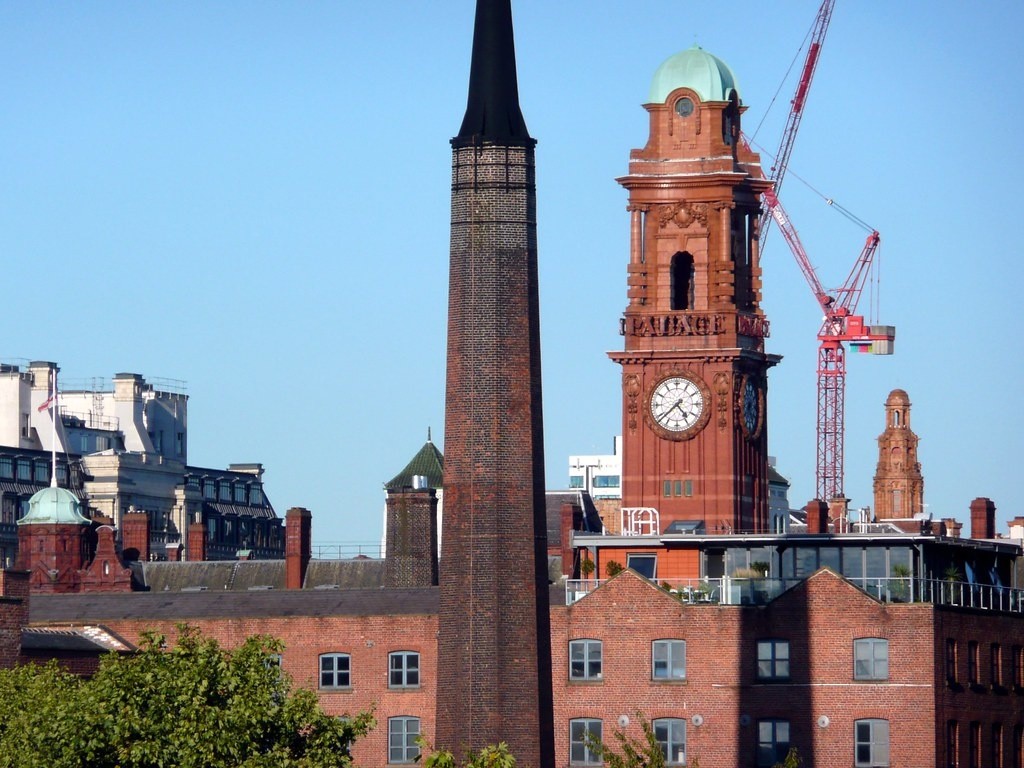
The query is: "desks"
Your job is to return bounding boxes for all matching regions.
[677,589,708,606]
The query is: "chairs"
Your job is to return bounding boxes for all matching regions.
[680,587,695,604]
[697,589,716,605]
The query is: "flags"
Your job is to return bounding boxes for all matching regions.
[37,393,55,414]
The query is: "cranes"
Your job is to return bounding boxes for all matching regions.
[737,0,897,533]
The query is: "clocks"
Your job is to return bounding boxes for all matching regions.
[742,375,762,438]
[642,368,710,441]
